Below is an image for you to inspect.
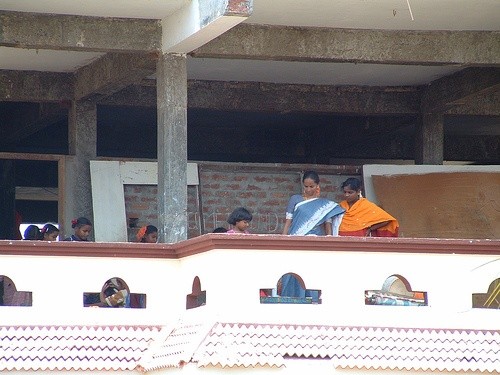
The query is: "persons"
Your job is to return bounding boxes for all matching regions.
[336,177,399,237]
[214,207,252,234]
[282,170,346,236]
[24,224,60,241]
[64,216,92,241]
[136,225,158,243]
